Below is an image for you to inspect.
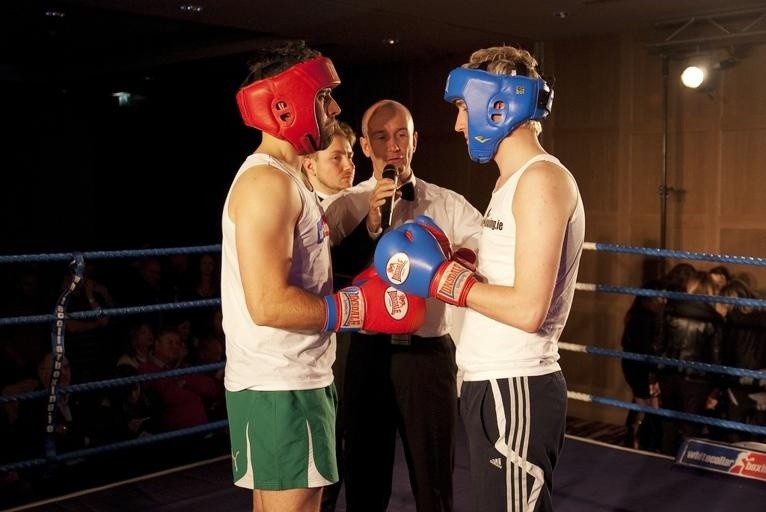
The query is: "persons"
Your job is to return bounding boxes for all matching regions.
[0,254,230,505]
[220,43,427,512]
[320,98,484,510]
[619,262,766,459]
[296,120,358,512]
[373,45,587,512]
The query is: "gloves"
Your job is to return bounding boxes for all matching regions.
[318,264,427,336]
[374,215,478,309]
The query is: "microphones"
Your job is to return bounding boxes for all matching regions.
[381,164,399,229]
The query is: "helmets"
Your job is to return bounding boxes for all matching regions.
[444,65,555,166]
[235,56,342,157]
